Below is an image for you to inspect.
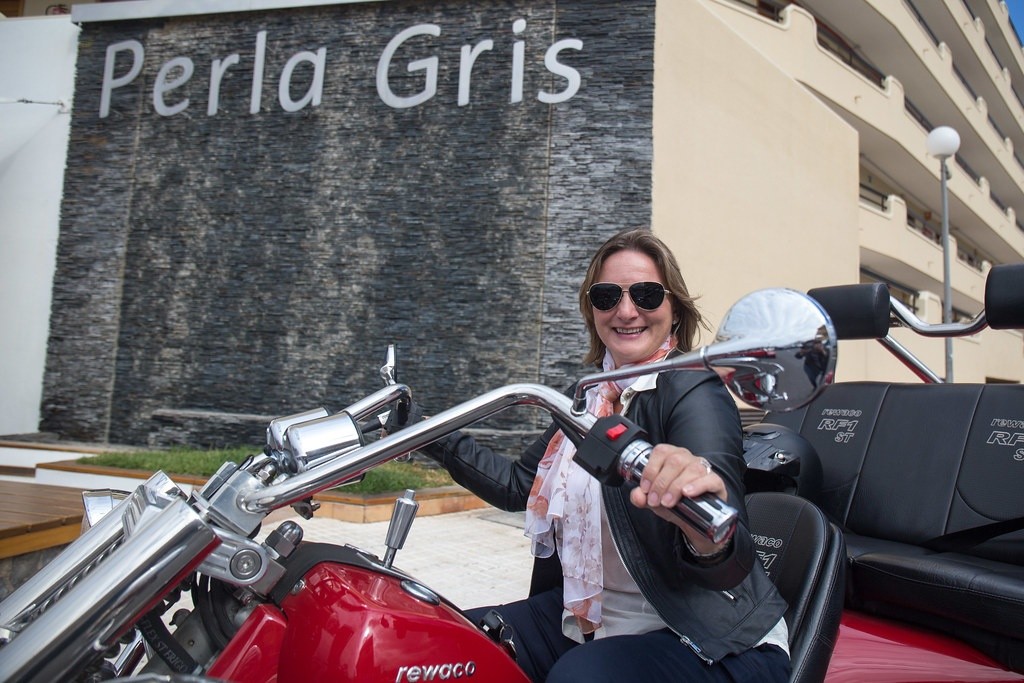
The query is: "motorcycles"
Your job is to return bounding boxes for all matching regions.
[0,263,1024,683]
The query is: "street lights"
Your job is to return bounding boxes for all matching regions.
[926,125,961,383]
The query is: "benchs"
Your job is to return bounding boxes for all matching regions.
[761,263,1024,641]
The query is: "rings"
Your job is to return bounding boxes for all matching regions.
[701,459,712,474]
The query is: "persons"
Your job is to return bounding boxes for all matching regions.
[794,324,829,388]
[381,226,791,683]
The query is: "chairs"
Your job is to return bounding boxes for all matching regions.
[741,491,849,683]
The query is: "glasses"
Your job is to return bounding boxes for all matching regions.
[586,280,673,312]
